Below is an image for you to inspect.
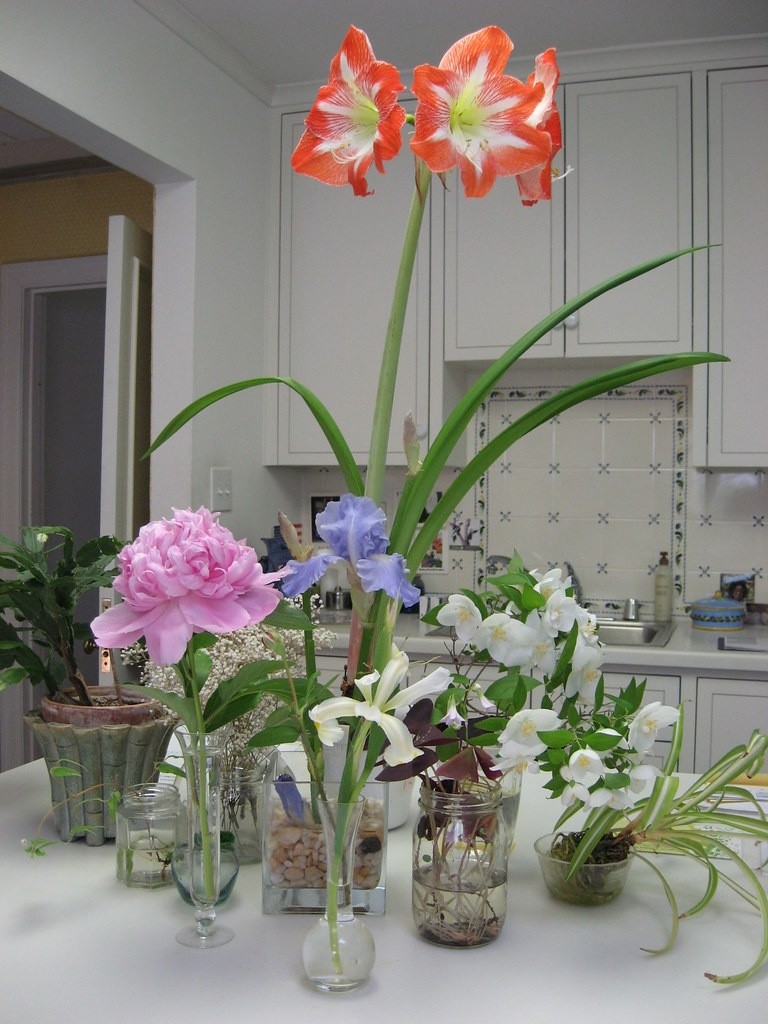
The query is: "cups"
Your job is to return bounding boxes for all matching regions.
[321,568,337,607]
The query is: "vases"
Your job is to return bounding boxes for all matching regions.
[171,718,528,996]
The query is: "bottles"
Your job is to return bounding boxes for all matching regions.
[401,575,425,613]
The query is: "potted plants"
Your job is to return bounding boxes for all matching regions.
[0,523,177,841]
[18,716,190,889]
[532,699,766,987]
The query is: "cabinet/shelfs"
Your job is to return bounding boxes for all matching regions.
[269,651,768,781]
[267,59,768,471]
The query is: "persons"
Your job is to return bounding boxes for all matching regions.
[728,580,749,602]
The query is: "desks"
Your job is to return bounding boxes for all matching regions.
[0,739,764,1024]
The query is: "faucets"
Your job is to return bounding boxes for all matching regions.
[486,556,581,603]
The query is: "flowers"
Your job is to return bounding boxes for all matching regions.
[136,17,735,755]
[261,624,452,973]
[88,505,316,904]
[385,558,679,810]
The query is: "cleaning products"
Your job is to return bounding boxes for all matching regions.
[654,552,673,622]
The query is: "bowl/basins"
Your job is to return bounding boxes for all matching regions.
[326,586,352,610]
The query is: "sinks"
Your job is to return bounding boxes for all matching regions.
[596,618,676,647]
